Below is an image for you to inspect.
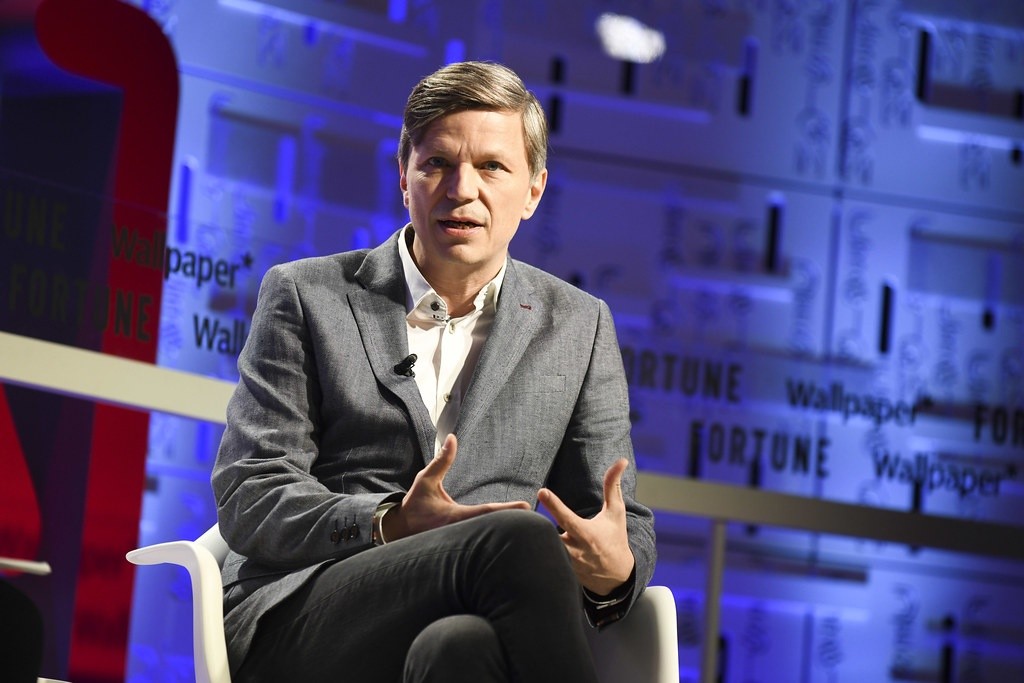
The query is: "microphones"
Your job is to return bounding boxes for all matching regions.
[394,354,417,379]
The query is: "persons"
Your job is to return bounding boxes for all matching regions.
[208,62,659,683]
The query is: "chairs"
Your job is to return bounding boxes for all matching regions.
[120,506,684,682]
